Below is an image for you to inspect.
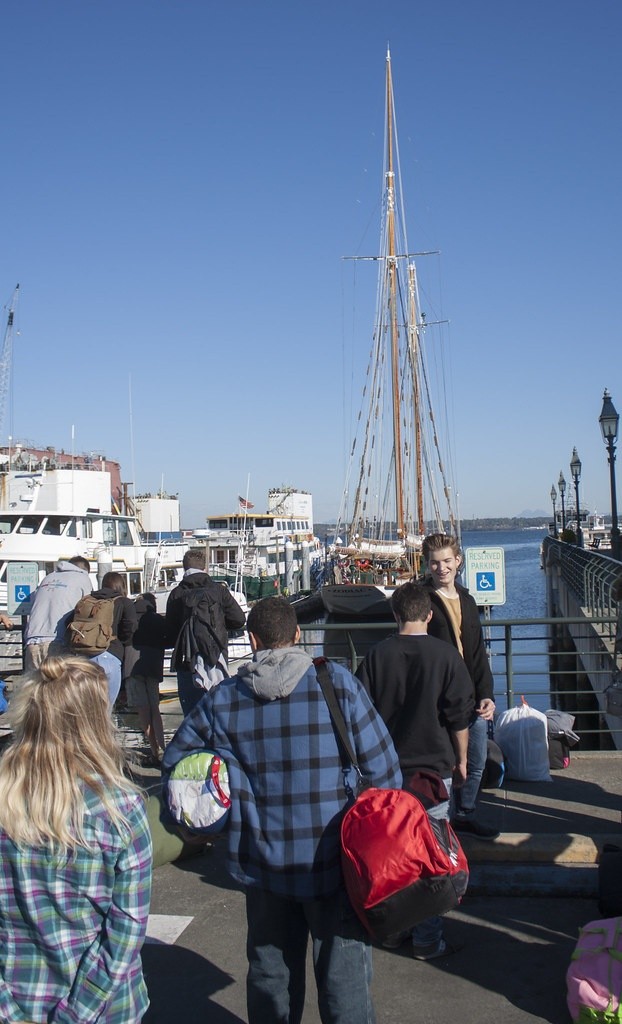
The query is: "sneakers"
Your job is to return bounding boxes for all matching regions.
[414,941,446,960]
[449,815,500,841]
[383,933,401,951]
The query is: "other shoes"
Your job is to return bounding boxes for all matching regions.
[141,754,162,770]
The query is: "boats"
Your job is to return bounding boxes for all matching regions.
[0,373,337,698]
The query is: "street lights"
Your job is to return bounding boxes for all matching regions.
[597,387,622,603]
[569,446,584,548]
[550,485,557,538]
[558,469,566,540]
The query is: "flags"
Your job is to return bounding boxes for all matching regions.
[240,496,254,509]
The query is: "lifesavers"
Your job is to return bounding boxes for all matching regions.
[338,559,350,568]
[274,579,278,588]
[355,559,369,568]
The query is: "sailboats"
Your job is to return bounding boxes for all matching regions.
[320,40,462,616]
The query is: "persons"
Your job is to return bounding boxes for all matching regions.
[354,583,474,959]
[0,654,153,1024]
[25,556,94,672]
[66,571,165,765]
[166,550,246,716]
[161,596,403,1024]
[423,534,500,839]
[0,614,14,632]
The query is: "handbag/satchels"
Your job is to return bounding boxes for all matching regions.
[548,732,571,769]
[566,916,622,1024]
[341,769,470,939]
[480,741,504,788]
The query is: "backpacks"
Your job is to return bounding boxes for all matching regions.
[182,587,216,634]
[67,596,124,656]
